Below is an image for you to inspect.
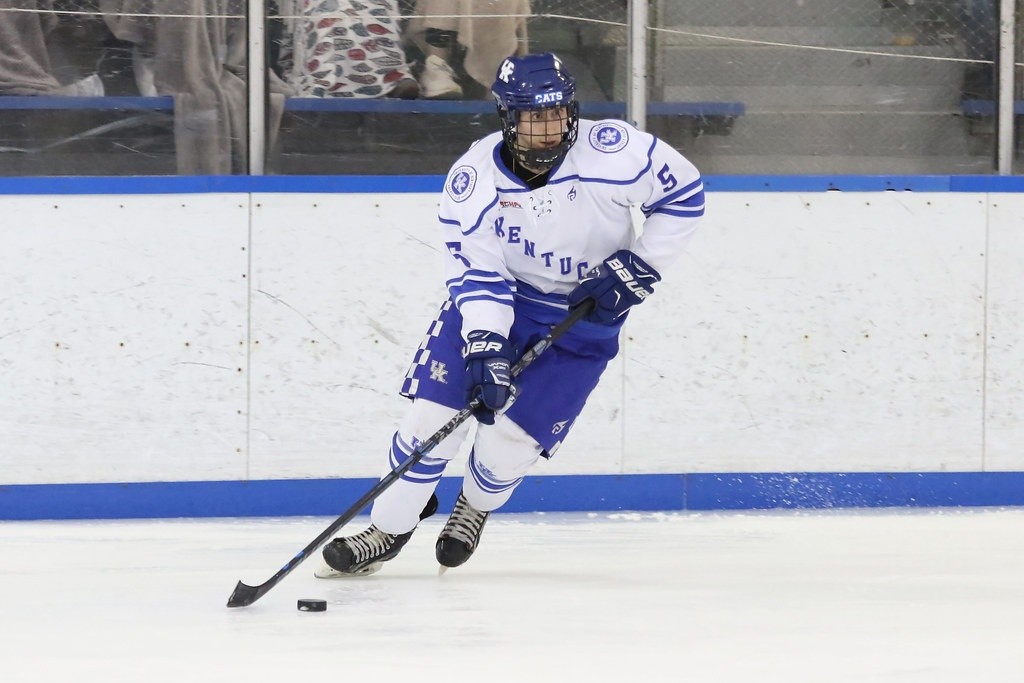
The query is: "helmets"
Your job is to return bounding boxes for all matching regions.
[491,51,581,171]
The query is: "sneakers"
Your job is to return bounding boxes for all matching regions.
[436,488,489,576]
[419,55,463,99]
[313,493,438,579]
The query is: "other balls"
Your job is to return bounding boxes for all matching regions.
[295,598,328,613]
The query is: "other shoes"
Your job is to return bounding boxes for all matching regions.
[425,28,488,98]
[392,72,421,99]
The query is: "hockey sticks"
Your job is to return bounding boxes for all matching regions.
[225,297,597,609]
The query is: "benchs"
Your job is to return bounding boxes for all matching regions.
[962,99,1024,127]
[0,93,747,156]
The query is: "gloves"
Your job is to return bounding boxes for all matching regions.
[461,330,517,424]
[569,250,661,326]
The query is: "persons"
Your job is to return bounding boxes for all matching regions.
[0,0,618,179]
[313,50,705,577]
